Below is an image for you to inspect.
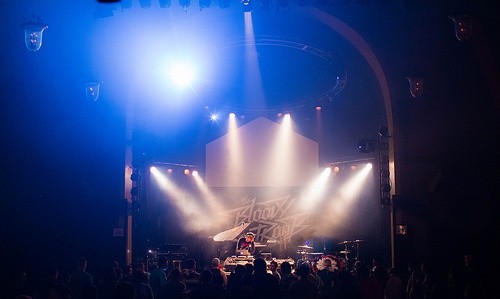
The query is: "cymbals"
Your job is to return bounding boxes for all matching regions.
[297,245,314,249]
[353,239,364,243]
[337,240,351,245]
[305,252,325,256]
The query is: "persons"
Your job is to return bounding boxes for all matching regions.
[235,232,255,255]
[1,254,500,299]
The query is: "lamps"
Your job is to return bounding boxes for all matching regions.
[93,0,308,19]
